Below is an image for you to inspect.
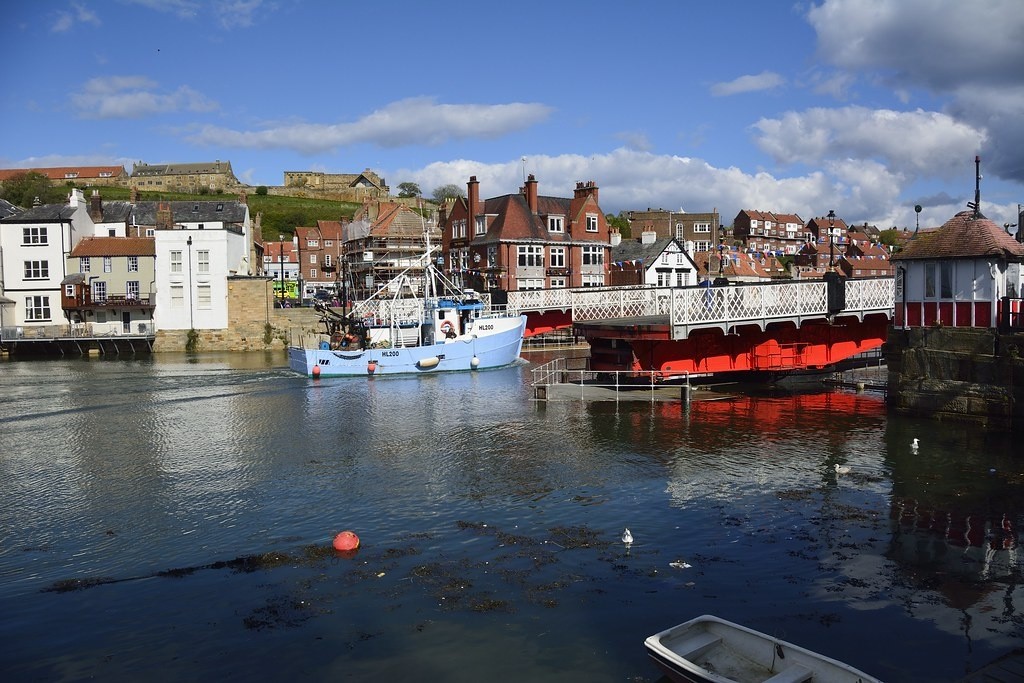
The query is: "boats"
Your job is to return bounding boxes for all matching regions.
[643,615,882,683]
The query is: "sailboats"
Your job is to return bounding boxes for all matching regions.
[287,177,527,376]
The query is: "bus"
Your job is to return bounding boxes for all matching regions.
[273,282,299,299]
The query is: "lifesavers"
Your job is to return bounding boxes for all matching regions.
[441,319,454,334]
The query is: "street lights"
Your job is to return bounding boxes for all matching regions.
[823,209,839,281]
[713,225,730,287]
[279,234,285,300]
